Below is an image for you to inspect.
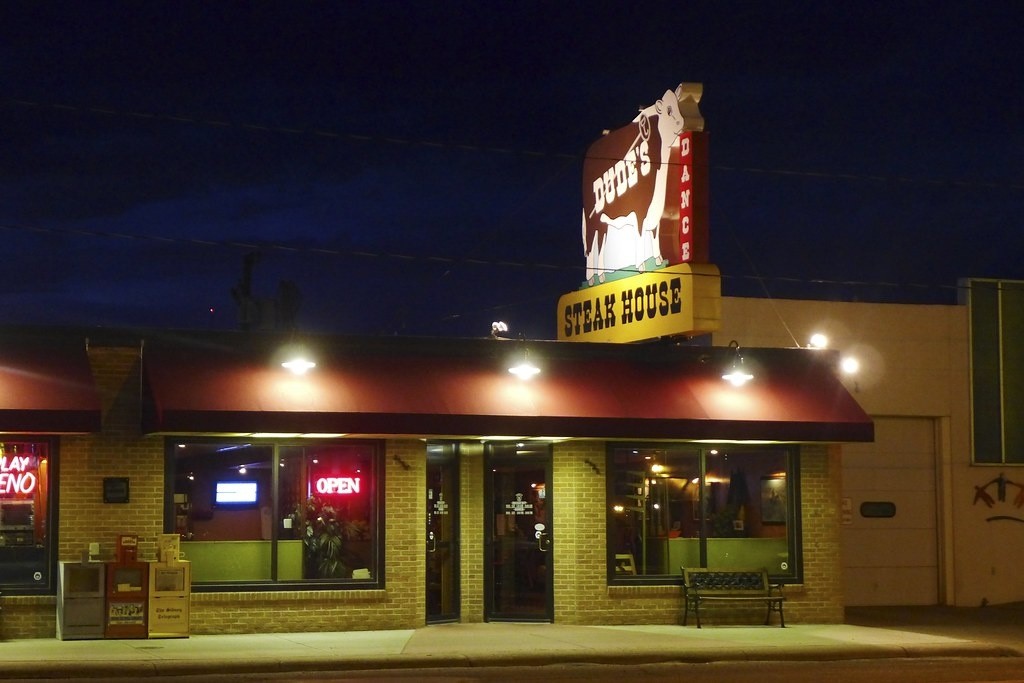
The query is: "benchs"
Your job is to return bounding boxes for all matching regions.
[680,565,785,628]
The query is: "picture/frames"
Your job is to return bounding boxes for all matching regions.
[760,476,786,524]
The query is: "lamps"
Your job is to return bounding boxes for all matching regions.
[281,324,316,374]
[490,321,540,381]
[721,340,754,386]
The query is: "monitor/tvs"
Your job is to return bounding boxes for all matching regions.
[213,479,259,509]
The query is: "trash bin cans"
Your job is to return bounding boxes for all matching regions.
[105,535,149,640]
[55,549,105,640]
[149,534,191,638]
